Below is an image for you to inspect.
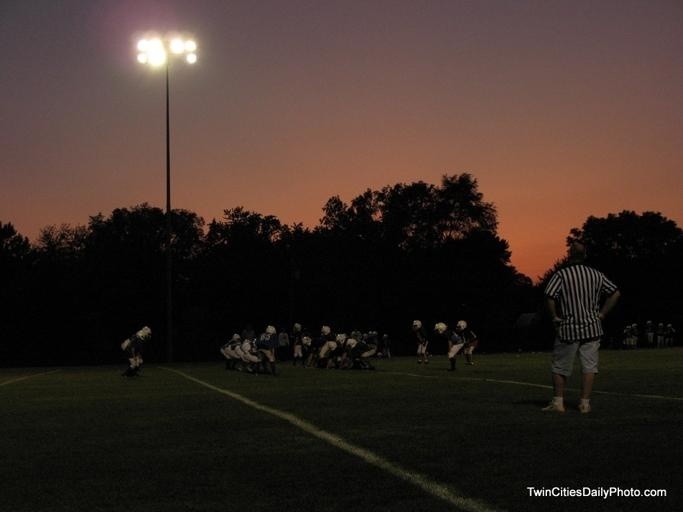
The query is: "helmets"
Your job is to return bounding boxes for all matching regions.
[136,326,151,343]
[348,339,358,349]
[336,335,347,343]
[435,323,446,333]
[321,326,331,335]
[266,326,277,335]
[233,334,241,342]
[413,321,422,329]
[456,321,467,330]
[295,323,302,330]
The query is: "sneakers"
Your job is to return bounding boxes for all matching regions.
[543,404,565,413]
[579,405,592,413]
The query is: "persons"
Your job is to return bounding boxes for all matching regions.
[455,319,479,366]
[218,322,392,377]
[433,322,464,371]
[118,326,154,380]
[411,319,429,366]
[540,242,620,415]
[621,321,675,350]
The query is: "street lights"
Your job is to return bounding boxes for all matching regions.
[135,33,200,362]
